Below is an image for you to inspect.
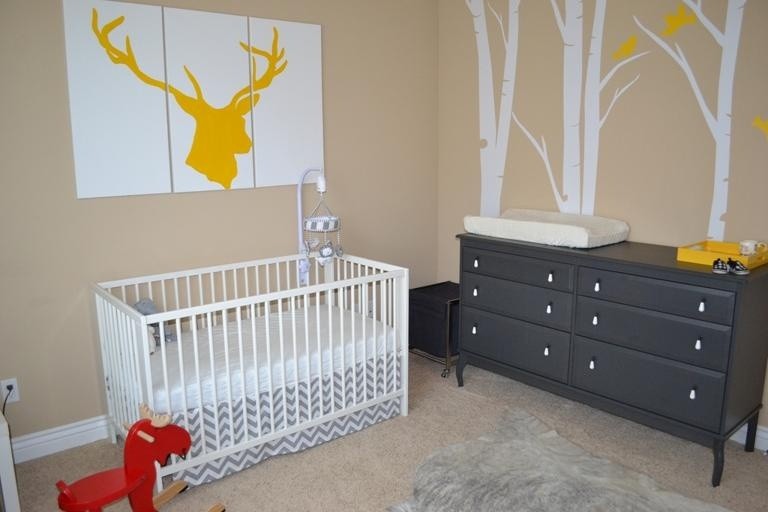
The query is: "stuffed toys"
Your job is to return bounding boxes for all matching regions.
[134,298,177,356]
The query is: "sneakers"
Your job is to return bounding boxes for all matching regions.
[712,258,751,275]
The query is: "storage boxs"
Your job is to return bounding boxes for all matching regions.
[409,281,460,358]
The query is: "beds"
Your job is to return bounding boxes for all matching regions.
[90,250,410,498]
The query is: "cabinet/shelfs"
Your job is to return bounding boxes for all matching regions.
[454,234,768,487]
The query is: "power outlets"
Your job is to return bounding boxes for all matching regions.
[0,377,19,404]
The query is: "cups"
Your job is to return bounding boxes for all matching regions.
[739,240,757,256]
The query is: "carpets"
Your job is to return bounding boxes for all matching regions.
[387,404,731,512]
